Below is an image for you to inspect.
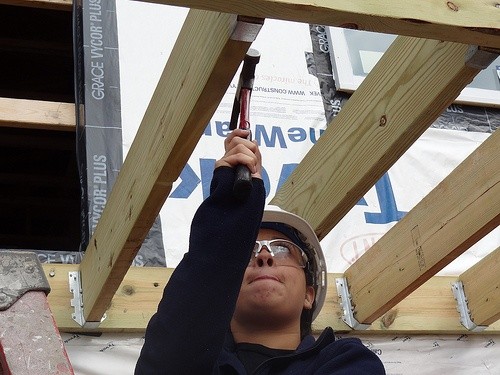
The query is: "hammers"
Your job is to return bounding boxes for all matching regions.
[229,49,263,188]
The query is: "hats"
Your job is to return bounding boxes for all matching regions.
[263,203,328,321]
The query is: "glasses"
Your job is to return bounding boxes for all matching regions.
[251,238,311,288]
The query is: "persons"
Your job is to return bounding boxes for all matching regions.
[134,129,386,375]
